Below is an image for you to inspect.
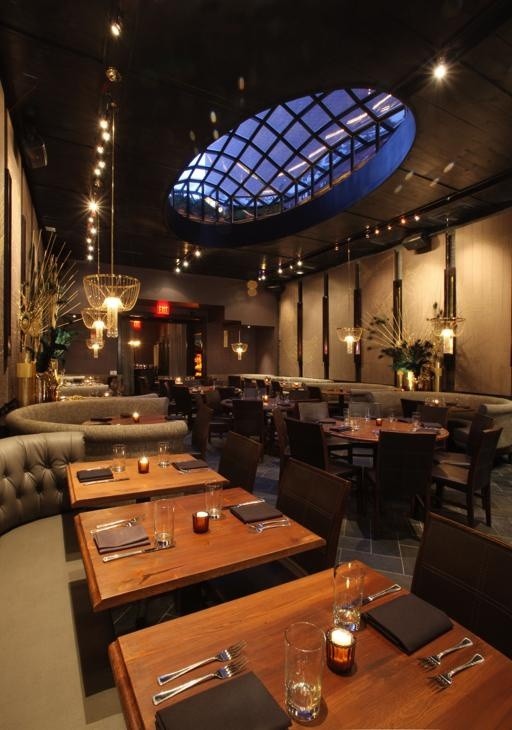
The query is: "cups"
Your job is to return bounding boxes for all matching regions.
[152,498,177,550]
[202,476,220,523]
[329,563,366,634]
[342,405,425,439]
[135,456,150,473]
[155,443,170,470]
[110,443,127,475]
[326,601,358,675]
[279,622,326,722]
[189,510,209,535]
[166,375,315,406]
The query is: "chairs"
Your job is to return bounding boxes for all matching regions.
[0,372,512,730]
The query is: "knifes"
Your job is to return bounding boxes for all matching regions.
[102,547,157,565]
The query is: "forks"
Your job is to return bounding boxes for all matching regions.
[354,582,401,608]
[157,638,248,687]
[415,637,487,691]
[150,655,248,708]
[242,516,294,535]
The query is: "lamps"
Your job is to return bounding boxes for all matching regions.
[81,201,116,338]
[428,212,466,357]
[85,334,108,359]
[230,329,250,363]
[81,98,141,339]
[336,235,364,357]
